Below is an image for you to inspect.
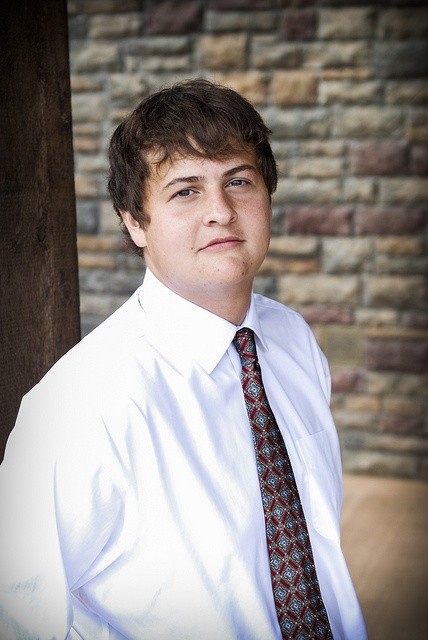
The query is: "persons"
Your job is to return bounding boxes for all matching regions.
[0,79,366,640]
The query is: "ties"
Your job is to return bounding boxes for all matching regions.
[232,328,333,639]
